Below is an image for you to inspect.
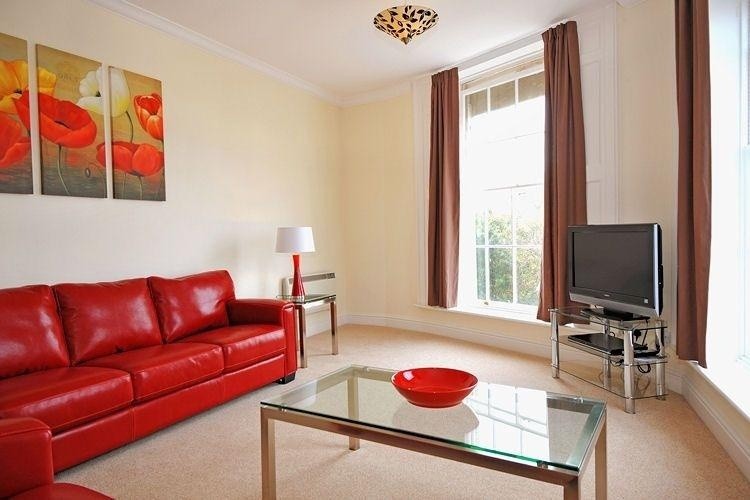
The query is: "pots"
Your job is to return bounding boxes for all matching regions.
[390,368,478,409]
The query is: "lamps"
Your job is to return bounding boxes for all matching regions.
[373,0,437,44]
[275,226,316,297]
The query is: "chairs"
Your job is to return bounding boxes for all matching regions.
[0,417,115,500]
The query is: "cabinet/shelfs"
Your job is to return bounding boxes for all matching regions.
[548,305,672,415]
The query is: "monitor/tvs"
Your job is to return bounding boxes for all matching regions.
[567,222,664,322]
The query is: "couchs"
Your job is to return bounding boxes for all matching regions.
[0,268,299,479]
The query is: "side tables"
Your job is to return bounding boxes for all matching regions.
[274,293,338,369]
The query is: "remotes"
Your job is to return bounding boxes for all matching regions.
[621,348,658,358]
